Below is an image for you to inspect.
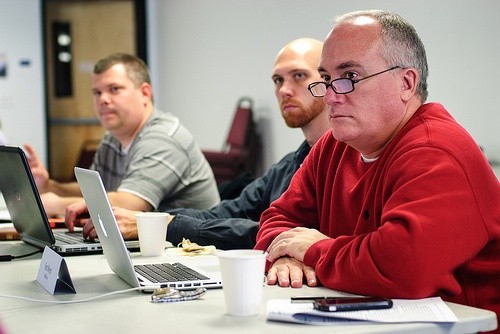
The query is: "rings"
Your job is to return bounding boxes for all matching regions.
[282,238,287,243]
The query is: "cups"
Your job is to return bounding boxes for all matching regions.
[217,250,267,315]
[135,213,170,256]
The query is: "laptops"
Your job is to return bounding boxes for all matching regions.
[75,167,223,291]
[0,147,173,254]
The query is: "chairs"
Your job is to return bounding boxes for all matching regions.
[201,94,263,183]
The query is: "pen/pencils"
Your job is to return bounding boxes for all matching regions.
[291,296,347,300]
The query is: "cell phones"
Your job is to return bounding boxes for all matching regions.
[316,296,393,311]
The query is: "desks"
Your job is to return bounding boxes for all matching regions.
[0,190,497,334]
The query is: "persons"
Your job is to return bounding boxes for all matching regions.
[24,53,220,218]
[64,38,332,251]
[254,8,500,334]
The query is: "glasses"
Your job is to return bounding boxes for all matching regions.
[308,66,398,97]
